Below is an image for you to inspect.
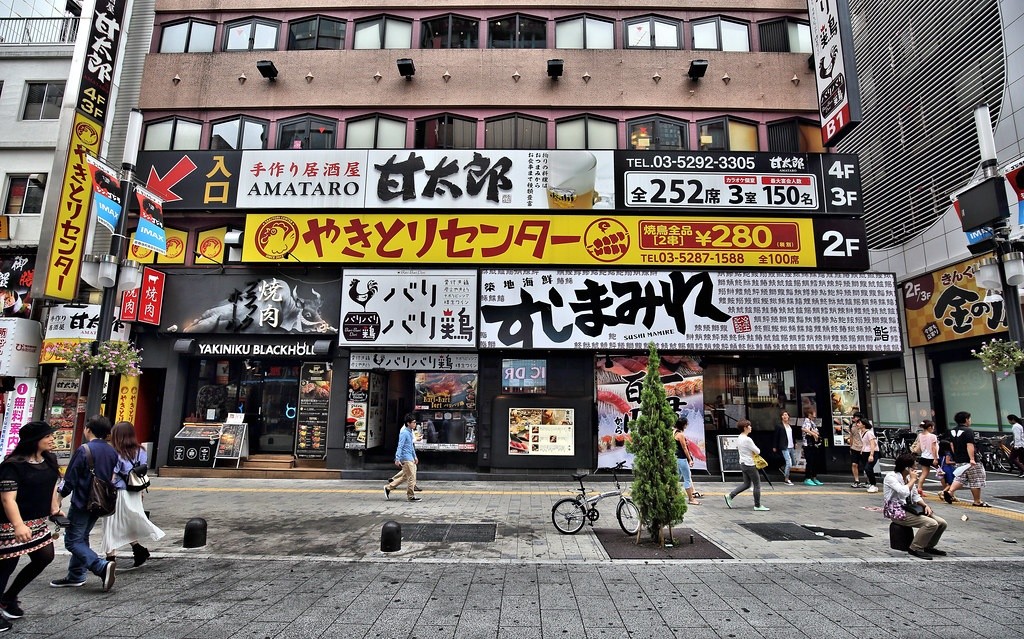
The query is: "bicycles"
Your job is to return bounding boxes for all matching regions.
[874,427,1015,474]
[550,460,642,536]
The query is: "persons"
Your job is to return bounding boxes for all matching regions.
[773,410,798,486]
[674,416,703,505]
[883,452,948,560]
[937,412,993,508]
[801,408,824,486]
[724,419,770,511]
[849,412,880,493]
[0,420,65,631]
[49,414,116,593]
[1007,414,1024,478]
[383,413,422,502]
[101,421,166,568]
[916,420,945,497]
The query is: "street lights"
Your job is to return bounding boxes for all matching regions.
[950,102,1024,420]
[80,144,168,449]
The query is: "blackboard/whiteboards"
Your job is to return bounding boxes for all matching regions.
[717,434,743,473]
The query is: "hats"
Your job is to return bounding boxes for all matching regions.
[18,421,57,442]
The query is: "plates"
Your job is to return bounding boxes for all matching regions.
[435,412,443,419]
[350,407,365,418]
[355,420,365,428]
[444,413,451,419]
[357,431,366,443]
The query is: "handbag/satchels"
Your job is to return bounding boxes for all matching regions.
[805,434,821,445]
[902,504,925,515]
[751,451,768,469]
[87,476,117,516]
[911,433,921,454]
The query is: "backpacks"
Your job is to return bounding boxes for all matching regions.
[123,448,150,493]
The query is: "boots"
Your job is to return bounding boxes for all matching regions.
[132,544,150,567]
[106,556,115,562]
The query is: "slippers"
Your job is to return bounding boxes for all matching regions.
[689,501,702,504]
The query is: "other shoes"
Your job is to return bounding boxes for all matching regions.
[785,480,795,486]
[724,494,733,509]
[692,492,703,498]
[414,485,422,491]
[388,478,396,489]
[384,485,390,500]
[754,505,770,511]
[814,479,824,486]
[919,489,992,507]
[804,480,817,486]
[407,497,423,502]
[908,547,932,560]
[925,547,947,556]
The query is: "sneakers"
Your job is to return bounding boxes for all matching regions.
[0,612,13,632]
[1,593,25,618]
[50,576,86,587]
[851,479,879,493]
[101,561,116,592]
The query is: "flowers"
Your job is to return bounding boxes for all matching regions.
[970,337,1024,382]
[47,336,147,376]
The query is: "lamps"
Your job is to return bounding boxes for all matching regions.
[173,337,197,354]
[256,60,279,81]
[193,250,226,273]
[313,339,333,355]
[397,58,416,80]
[284,252,305,268]
[688,60,708,81]
[547,59,564,79]
[605,353,614,366]
